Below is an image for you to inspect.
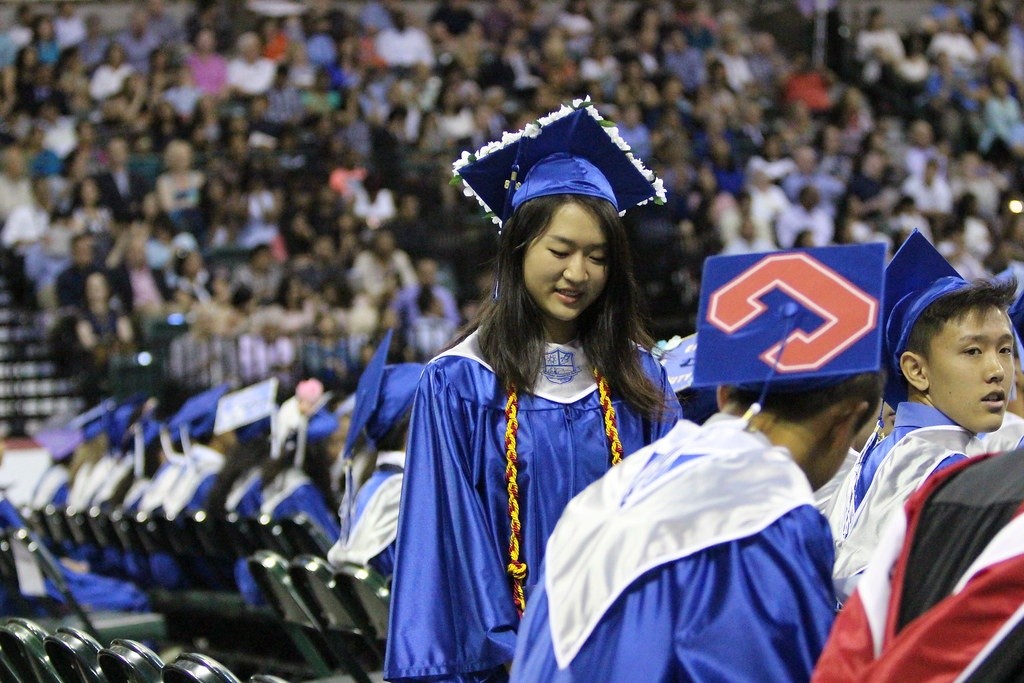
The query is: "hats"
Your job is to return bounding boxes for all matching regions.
[109,406,132,447]
[1008,289,1024,373]
[70,396,116,442]
[166,382,227,478]
[341,327,425,545]
[830,226,970,544]
[658,330,718,426]
[453,94,668,297]
[214,377,281,445]
[127,417,162,479]
[272,377,338,469]
[691,241,886,429]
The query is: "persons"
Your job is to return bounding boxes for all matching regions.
[385,93,680,683]
[0,0,1024,683]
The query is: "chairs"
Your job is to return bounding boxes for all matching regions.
[0,504,391,683]
